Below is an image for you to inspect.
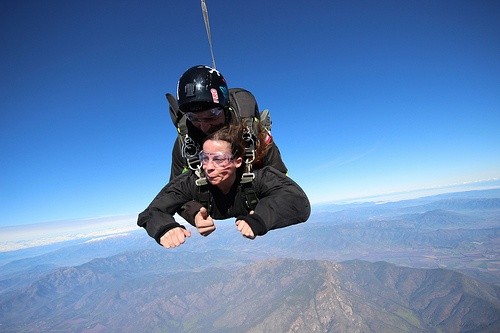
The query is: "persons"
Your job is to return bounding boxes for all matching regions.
[169,64,289,237]
[137,122,312,248]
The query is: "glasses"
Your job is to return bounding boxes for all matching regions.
[199,149,240,163]
[186,109,227,123]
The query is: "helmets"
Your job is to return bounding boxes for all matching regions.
[177,64,231,114]
